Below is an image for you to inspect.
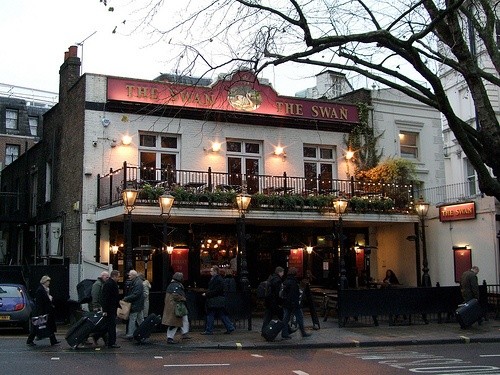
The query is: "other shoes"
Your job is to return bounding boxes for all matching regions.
[51,341,60,345]
[289,329,297,334]
[303,333,310,337]
[108,344,120,348]
[121,335,132,340]
[200,331,213,335]
[182,336,191,339]
[167,339,176,343]
[282,335,291,338]
[224,329,234,334]
[27,341,36,346]
[313,324,319,329]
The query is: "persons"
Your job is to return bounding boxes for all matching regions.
[119,270,151,339]
[161,272,192,345]
[25,277,61,346]
[383,269,401,285]
[259,267,312,338]
[90,269,122,348]
[460,266,488,327]
[305,269,317,286]
[200,266,235,335]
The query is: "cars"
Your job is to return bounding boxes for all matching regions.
[0,284,36,334]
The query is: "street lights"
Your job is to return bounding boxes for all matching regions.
[415,194,434,287]
[235,187,252,304]
[120,182,138,285]
[332,193,349,291]
[159,186,175,291]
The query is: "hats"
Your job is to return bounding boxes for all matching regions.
[39,276,51,284]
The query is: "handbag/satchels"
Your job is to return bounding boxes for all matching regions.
[175,301,189,317]
[117,300,131,319]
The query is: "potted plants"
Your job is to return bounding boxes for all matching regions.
[139,179,394,218]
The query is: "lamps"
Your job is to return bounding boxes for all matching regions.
[354,242,362,256]
[452,245,470,249]
[111,242,119,254]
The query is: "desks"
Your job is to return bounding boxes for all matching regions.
[308,284,338,321]
[273,186,294,195]
[182,182,206,190]
[361,191,380,200]
[322,189,339,196]
[219,183,241,191]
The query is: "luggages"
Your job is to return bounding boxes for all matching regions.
[453,298,483,330]
[133,313,162,343]
[65,314,104,350]
[261,318,284,341]
